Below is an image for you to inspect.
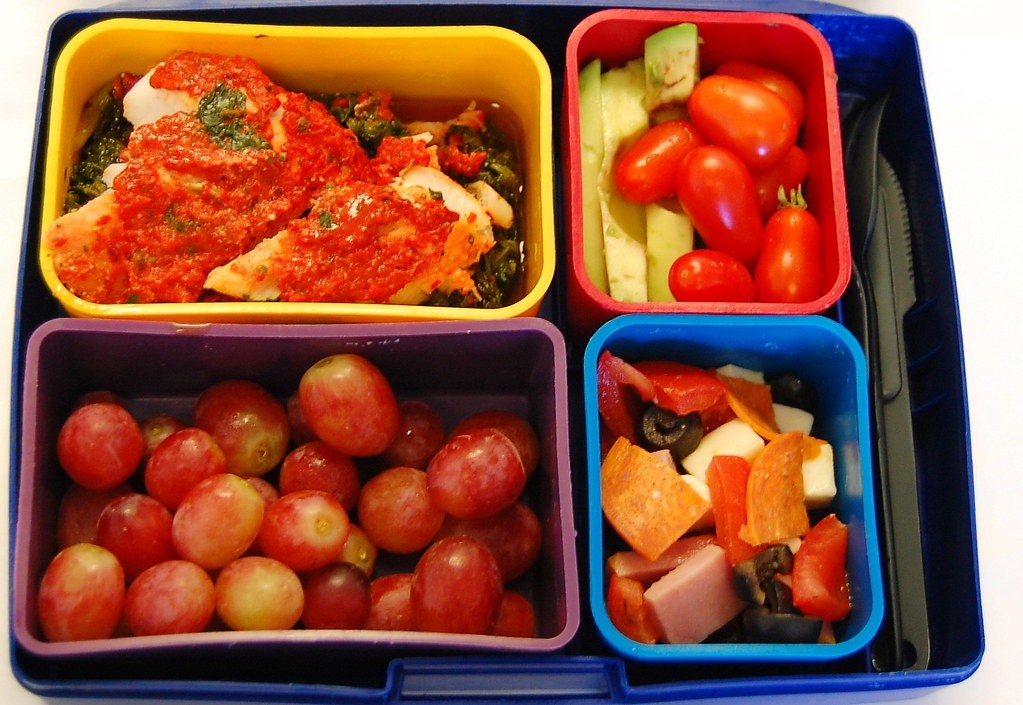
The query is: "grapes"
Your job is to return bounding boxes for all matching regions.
[34,350,539,644]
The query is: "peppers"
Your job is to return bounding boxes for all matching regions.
[597,350,853,647]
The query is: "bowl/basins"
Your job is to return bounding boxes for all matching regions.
[562,10,852,315]
[583,315,886,662]
[38,18,556,324]
[11,319,579,655]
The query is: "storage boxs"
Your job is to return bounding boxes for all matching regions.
[10,8,988,705]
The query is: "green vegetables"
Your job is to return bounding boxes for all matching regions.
[69,88,525,312]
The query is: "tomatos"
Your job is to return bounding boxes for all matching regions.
[614,62,820,306]
